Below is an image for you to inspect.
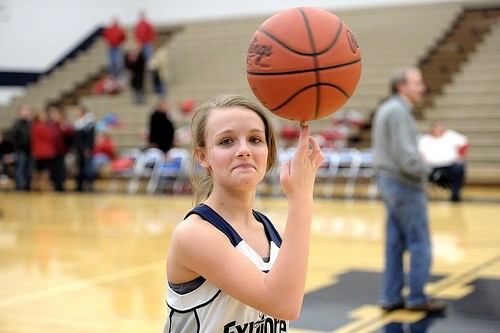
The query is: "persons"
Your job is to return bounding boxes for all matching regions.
[372,66,448,313]
[418,120,470,202]
[103,8,170,106]
[166,94,324,333]
[11,103,97,193]
[147,98,198,154]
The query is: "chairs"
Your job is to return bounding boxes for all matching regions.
[118,148,380,202]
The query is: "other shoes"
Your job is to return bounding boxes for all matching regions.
[381,297,404,309]
[405,299,447,311]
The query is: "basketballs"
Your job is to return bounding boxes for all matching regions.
[247,7,362,121]
